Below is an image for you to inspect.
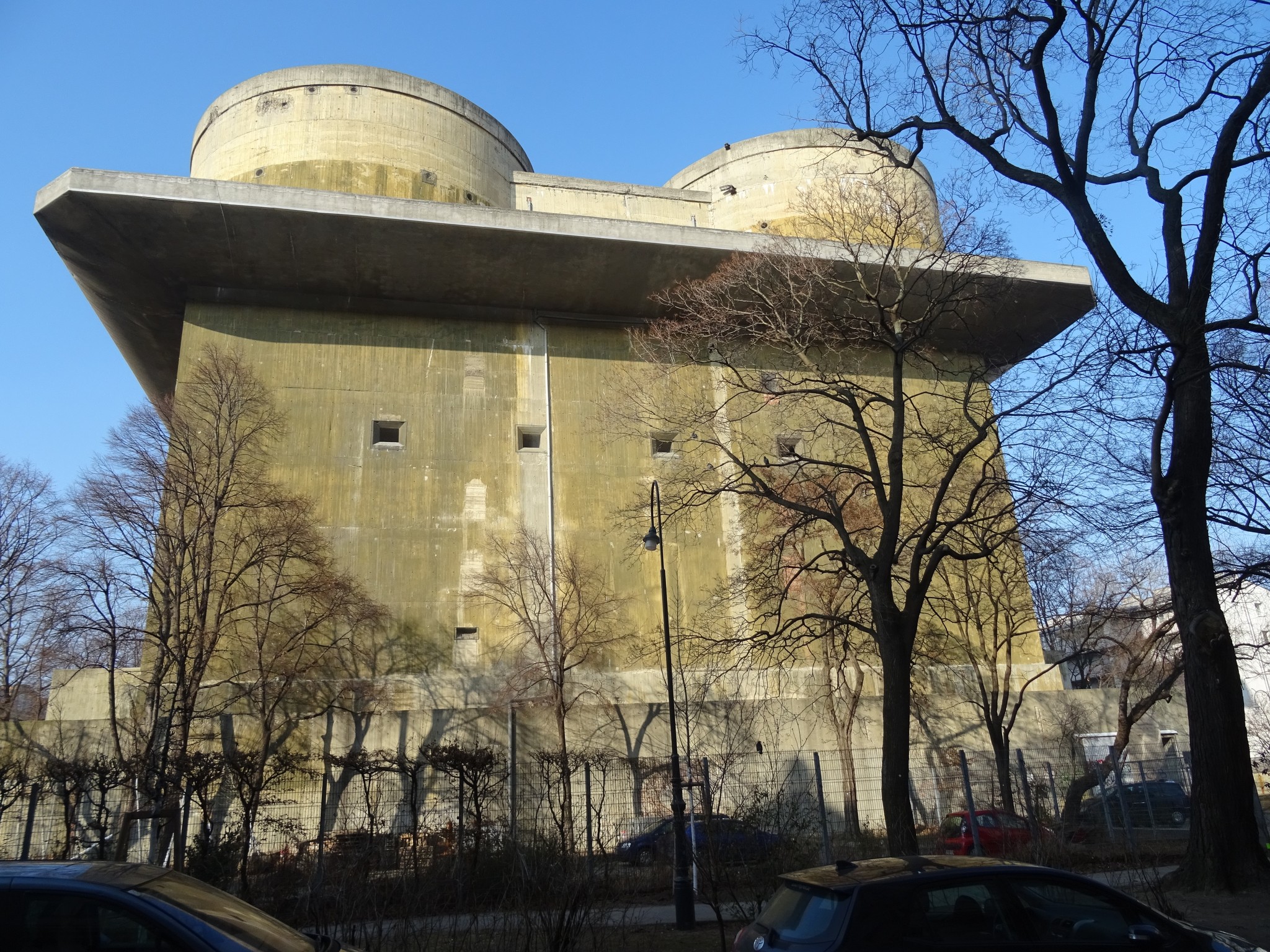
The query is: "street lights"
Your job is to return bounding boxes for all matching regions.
[642,480,697,932]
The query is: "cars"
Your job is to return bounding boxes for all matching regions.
[0,859,364,952]
[936,809,1058,858]
[663,818,783,866]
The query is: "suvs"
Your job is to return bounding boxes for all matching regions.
[734,855,1270,952]
[1078,780,1191,827]
[614,813,737,867]
